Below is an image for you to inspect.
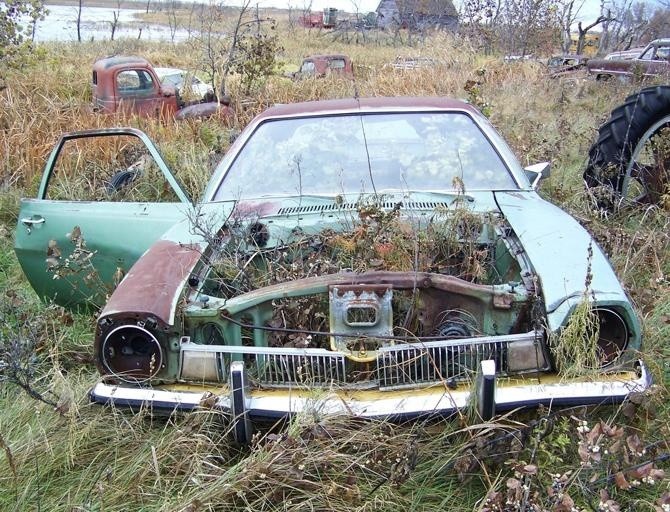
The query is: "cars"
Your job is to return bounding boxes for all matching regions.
[13,98,648,429]
[587,38,670,88]
